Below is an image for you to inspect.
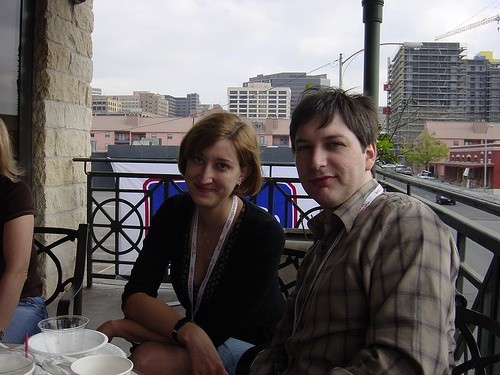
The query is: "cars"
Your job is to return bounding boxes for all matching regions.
[436,181,456,205]
[381,164,435,178]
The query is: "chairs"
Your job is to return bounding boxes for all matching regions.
[448,299,500,375]
[131,244,306,369]
[31,223,89,329]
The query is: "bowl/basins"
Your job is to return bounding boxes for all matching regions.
[70,356,134,375]
[0,349,35,375]
[27,329,108,356]
[37,315,89,353]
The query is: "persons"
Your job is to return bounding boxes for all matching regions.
[0,115,52,344]
[97,113,288,375]
[247,87,461,375]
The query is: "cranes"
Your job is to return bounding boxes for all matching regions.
[432,0,500,40]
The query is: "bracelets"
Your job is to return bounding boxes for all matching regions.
[0,330,6,341]
[171,317,192,342]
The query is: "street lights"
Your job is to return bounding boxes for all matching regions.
[339,41,424,90]
[474,124,493,192]
